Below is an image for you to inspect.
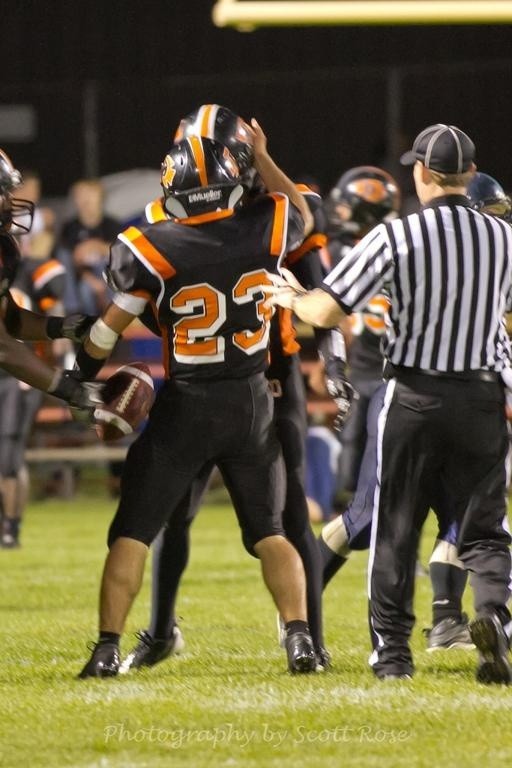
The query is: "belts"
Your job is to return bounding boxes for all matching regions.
[390,365,501,385]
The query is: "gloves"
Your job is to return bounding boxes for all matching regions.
[327,380,357,431]
[62,308,97,343]
[48,368,106,409]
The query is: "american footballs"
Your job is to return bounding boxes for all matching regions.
[94,360,154,443]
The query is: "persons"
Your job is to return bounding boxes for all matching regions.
[1,170,66,548]
[259,125,512,684]
[2,152,156,439]
[51,180,130,340]
[72,104,315,676]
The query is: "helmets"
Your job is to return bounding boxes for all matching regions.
[160,137,245,225]
[467,172,512,218]
[330,166,403,223]
[0,151,36,238]
[172,105,259,192]
[288,180,325,215]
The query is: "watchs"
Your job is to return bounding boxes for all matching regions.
[292,293,303,311]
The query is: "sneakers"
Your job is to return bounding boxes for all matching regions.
[380,669,414,682]
[284,632,316,675]
[1,529,21,547]
[315,645,336,671]
[120,628,185,673]
[76,646,122,677]
[467,611,512,684]
[427,624,482,652]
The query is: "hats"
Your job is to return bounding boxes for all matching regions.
[401,123,476,175]
